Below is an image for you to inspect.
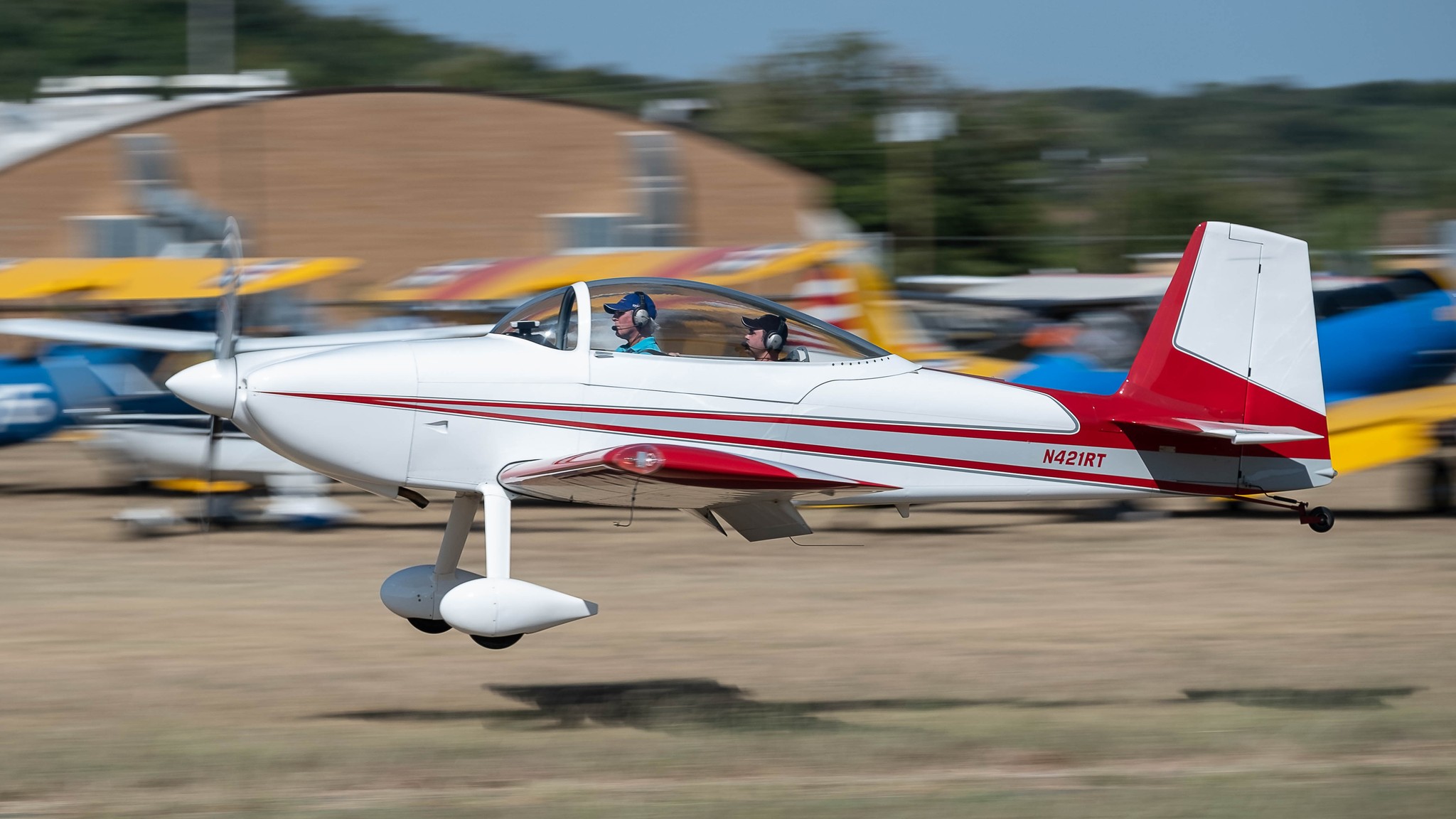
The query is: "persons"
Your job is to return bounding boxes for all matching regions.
[604,292,662,356]
[740,312,788,362]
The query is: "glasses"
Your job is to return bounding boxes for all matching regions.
[614,308,634,318]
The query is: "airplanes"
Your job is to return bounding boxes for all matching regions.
[165,208,1335,651]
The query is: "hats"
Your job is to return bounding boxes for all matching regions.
[740,314,788,340]
[602,291,657,320]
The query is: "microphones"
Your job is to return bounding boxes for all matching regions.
[612,322,642,331]
[741,342,773,351]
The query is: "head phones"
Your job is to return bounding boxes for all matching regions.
[763,316,787,353]
[633,291,650,328]
